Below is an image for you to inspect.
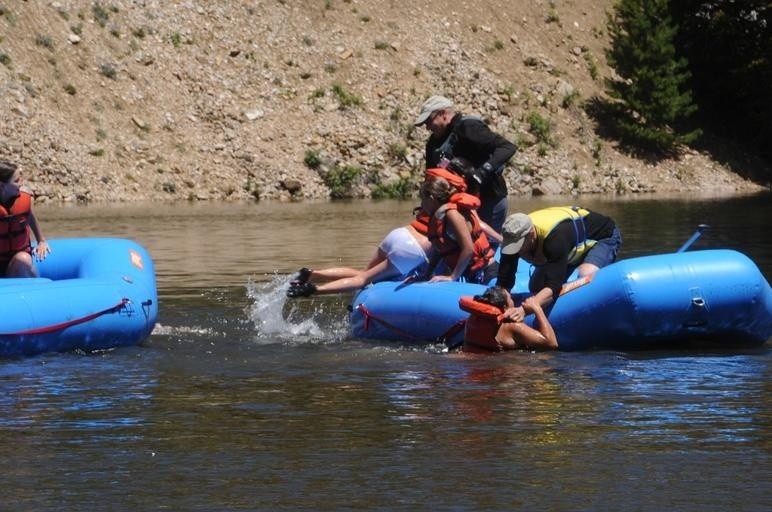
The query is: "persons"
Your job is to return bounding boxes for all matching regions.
[404,177,498,286]
[496,205,621,297]
[416,95,518,240]
[288,158,469,297]
[463,286,559,353]
[0,161,50,280]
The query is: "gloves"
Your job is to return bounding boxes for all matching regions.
[467,168,488,193]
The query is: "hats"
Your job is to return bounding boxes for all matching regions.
[500,213,532,254]
[415,96,453,126]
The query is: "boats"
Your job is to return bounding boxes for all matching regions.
[347,249,772,349]
[0,237,158,360]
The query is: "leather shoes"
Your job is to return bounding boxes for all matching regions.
[286,282,314,297]
[290,268,313,282]
